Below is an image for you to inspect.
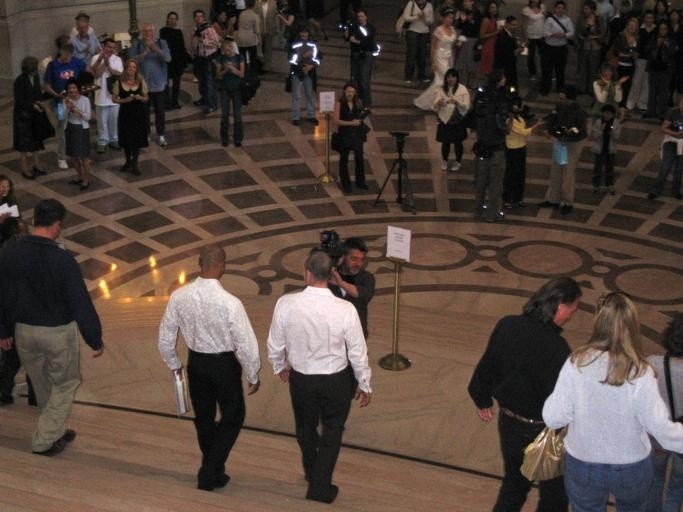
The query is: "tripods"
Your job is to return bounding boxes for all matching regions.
[372,131,421,215]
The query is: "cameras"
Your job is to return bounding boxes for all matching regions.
[356,106,371,120]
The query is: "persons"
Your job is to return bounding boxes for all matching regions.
[0,217,39,407]
[1,176,23,220]
[464,276,584,510]
[328,237,376,343]
[643,313,683,511]
[264,250,372,500]
[157,243,261,492]
[338,2,680,119]
[0,198,105,458]
[14,1,330,189]
[433,68,472,173]
[542,291,683,511]
[328,82,374,190]
[472,90,680,220]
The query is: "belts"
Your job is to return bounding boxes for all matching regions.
[502,408,544,424]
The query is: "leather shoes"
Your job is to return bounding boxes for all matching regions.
[292,118,318,126]
[305,485,338,503]
[22,160,89,190]
[33,429,75,455]
[223,142,242,146]
[167,102,215,113]
[198,474,230,491]
[592,188,615,196]
[648,193,683,199]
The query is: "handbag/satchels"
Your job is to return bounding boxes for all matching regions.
[519,427,567,481]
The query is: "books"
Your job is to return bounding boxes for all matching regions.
[172,368,191,417]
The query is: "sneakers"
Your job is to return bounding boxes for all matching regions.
[96,136,167,176]
[345,184,369,192]
[440,160,460,171]
[474,200,574,222]
